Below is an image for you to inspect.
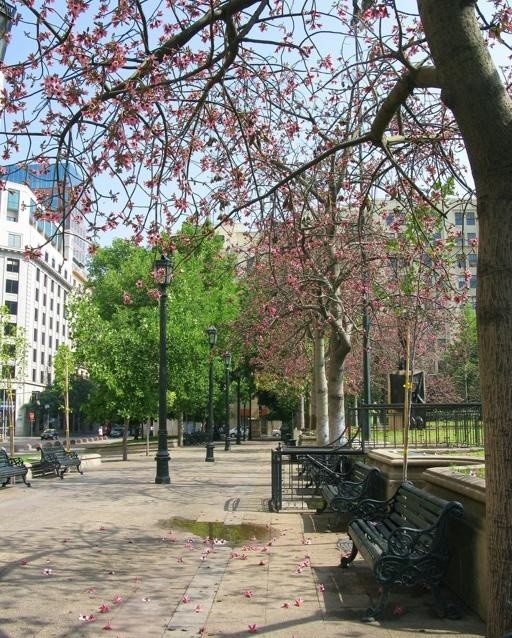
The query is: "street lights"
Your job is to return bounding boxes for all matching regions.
[205,324,256,462]
[154,254,175,484]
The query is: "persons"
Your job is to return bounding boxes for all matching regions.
[99,425,104,436]
[104,422,110,436]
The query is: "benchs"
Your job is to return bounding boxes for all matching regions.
[0,443,85,490]
[296,447,468,623]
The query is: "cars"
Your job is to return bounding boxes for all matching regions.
[272,430,282,437]
[110,426,132,437]
[40,428,60,440]
